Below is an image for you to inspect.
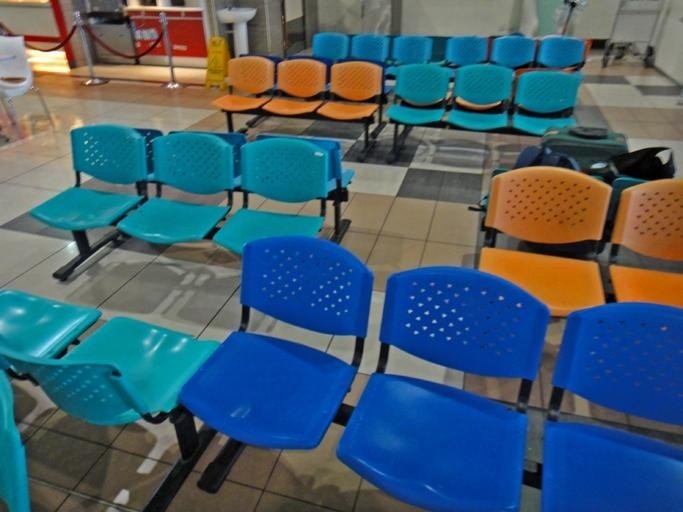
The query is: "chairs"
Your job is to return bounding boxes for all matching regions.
[178,236,371,489]
[0,291,104,382]
[30,123,356,286]
[3,314,225,509]
[543,302,682,512]
[2,34,55,138]
[335,267,550,510]
[3,367,30,512]
[470,163,681,322]
[210,27,590,166]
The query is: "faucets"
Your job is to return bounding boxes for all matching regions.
[228,4,239,11]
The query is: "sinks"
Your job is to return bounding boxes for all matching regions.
[216,7,258,24]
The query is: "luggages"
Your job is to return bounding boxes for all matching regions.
[540,126,629,168]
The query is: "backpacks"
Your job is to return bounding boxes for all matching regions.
[468,147,581,216]
